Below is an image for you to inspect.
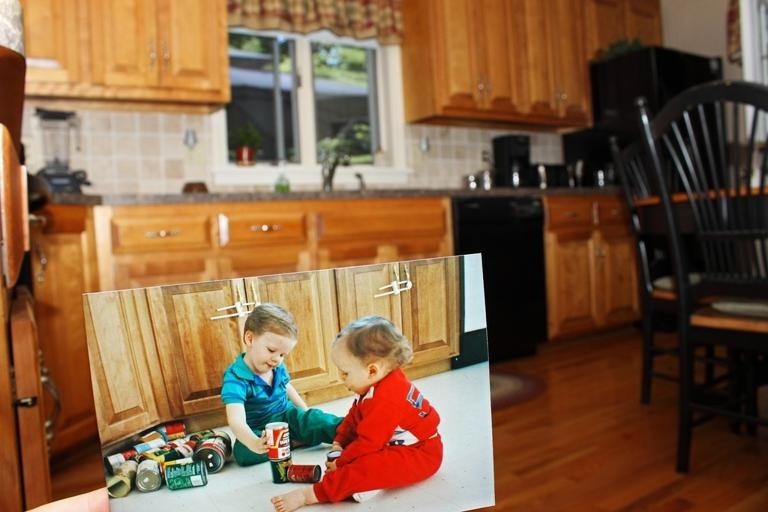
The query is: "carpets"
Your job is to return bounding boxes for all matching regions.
[487,367,547,415]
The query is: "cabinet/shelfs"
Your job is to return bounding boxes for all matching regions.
[400,1,525,129]
[589,2,666,67]
[336,257,464,399]
[1,281,58,512]
[521,2,591,135]
[91,204,310,287]
[29,202,111,458]
[78,287,175,448]
[538,190,642,344]
[78,1,233,118]
[20,1,79,111]
[311,197,453,268]
[143,267,338,433]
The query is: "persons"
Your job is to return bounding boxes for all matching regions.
[219,304,345,467]
[269,314,444,512]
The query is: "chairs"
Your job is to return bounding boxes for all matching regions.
[632,76,768,475]
[608,129,745,434]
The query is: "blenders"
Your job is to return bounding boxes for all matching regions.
[34,105,90,195]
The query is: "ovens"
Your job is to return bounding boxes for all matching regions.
[447,194,548,362]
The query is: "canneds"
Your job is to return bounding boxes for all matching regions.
[288,464,321,482]
[270,457,293,484]
[326,450,341,463]
[104,423,231,498]
[265,422,290,459]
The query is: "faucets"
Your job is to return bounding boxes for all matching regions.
[319,146,354,193]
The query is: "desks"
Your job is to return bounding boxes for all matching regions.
[632,188,767,436]
